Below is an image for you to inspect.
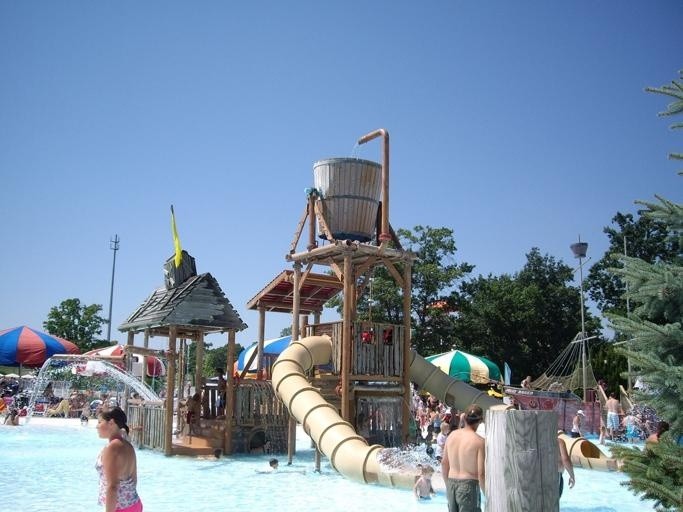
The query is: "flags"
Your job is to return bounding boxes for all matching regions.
[171,205,183,269]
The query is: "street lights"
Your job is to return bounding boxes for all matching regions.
[569,234,592,404]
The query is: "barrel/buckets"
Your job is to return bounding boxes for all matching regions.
[313,156,384,241]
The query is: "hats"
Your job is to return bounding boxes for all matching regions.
[463,404,483,422]
[577,410,586,417]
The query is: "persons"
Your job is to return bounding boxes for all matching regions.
[213,448,223,460]
[598,379,605,391]
[604,392,621,437]
[620,412,642,443]
[412,391,486,512]
[520,376,533,389]
[570,409,586,439]
[647,421,669,442]
[94,406,143,512]
[269,458,278,467]
[0,376,143,426]
[557,439,575,499]
[357,409,378,446]
[215,368,227,419]
[186,394,201,424]
